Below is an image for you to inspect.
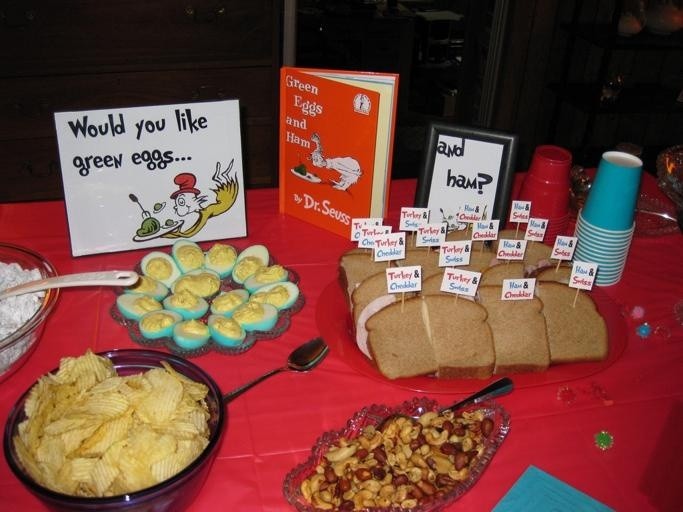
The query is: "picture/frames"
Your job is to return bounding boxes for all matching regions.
[405,118,520,241]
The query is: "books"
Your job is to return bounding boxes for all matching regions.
[489,463,613,512]
[276,61,403,240]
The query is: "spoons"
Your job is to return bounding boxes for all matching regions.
[224,339,327,404]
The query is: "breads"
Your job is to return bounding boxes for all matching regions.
[339,230,607,380]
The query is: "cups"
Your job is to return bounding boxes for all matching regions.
[514,145,641,287]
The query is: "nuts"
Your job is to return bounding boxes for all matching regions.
[301,409,494,511]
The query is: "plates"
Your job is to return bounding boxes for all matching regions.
[283,397,509,512]
[312,269,628,397]
[107,244,301,361]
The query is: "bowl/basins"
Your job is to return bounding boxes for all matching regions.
[1,240,58,383]
[2,350,226,511]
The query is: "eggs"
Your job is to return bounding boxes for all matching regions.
[117,239,299,349]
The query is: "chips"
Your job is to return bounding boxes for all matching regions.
[13,350,210,497]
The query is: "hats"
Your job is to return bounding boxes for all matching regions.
[169,172,202,200]
